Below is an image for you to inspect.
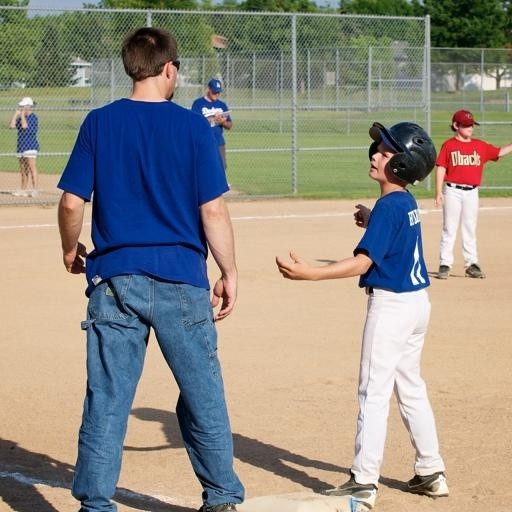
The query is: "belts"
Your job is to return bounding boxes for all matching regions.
[447,183,477,191]
[364,286,373,295]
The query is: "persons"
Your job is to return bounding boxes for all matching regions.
[274,122,449,510]
[435,109,512,278]
[60,27,244,512]
[9,97,39,197]
[192,80,233,189]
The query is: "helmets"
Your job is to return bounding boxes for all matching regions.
[368,121,438,186]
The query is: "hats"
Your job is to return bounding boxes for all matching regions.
[451,110,478,127]
[19,97,35,108]
[208,79,223,94]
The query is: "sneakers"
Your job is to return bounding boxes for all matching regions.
[464,264,485,279]
[438,265,451,279]
[12,189,41,198]
[197,503,238,512]
[400,473,450,498]
[318,477,378,510]
